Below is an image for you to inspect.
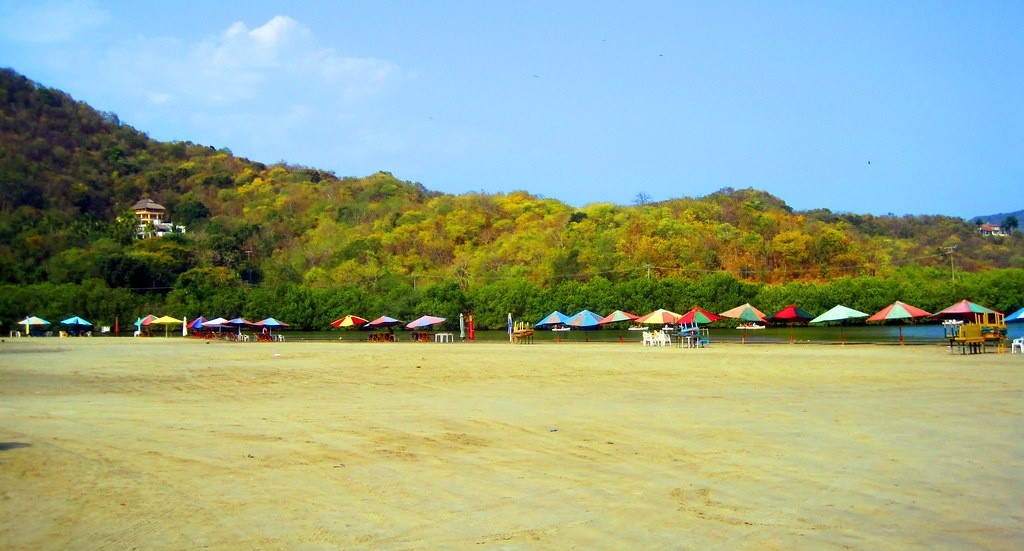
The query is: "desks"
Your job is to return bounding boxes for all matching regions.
[435,333,454,343]
[511,321,534,344]
[671,319,710,349]
[941,313,1008,356]
[390,335,399,342]
[134,330,143,337]
[10,330,20,337]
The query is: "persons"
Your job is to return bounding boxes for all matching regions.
[262,324,268,335]
[388,326,394,341]
[409,332,418,342]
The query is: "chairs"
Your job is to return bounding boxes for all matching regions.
[642,330,671,347]
[1011,336,1024,354]
[192,330,285,342]
[367,332,393,342]
[415,332,431,342]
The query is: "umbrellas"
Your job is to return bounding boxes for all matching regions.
[114,314,119,336]
[468,314,475,340]
[60,316,93,336]
[534,299,1024,346]
[330,313,447,340]
[507,312,512,342]
[16,315,51,336]
[459,312,467,342]
[134,315,291,339]
